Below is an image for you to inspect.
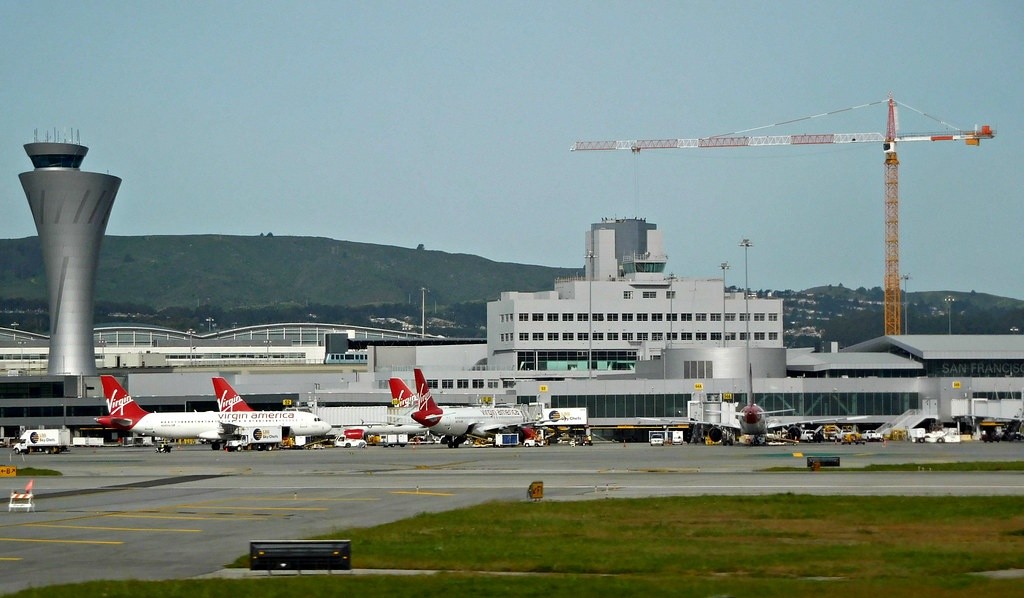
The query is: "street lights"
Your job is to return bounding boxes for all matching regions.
[205,317,215,332]
[900,272,912,334]
[737,238,753,363]
[98,337,107,370]
[943,295,960,335]
[716,260,732,288]
[419,287,431,339]
[662,271,679,348]
[583,249,598,380]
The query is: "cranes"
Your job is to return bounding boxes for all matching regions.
[568,90,999,337]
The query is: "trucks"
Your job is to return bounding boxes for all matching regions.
[671,430,684,445]
[12,429,71,454]
[333,434,367,448]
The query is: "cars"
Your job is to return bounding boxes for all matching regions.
[862,429,883,442]
[650,433,665,446]
[799,430,814,443]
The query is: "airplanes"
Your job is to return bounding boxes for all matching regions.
[92,374,333,452]
[337,368,564,449]
[704,362,844,446]
